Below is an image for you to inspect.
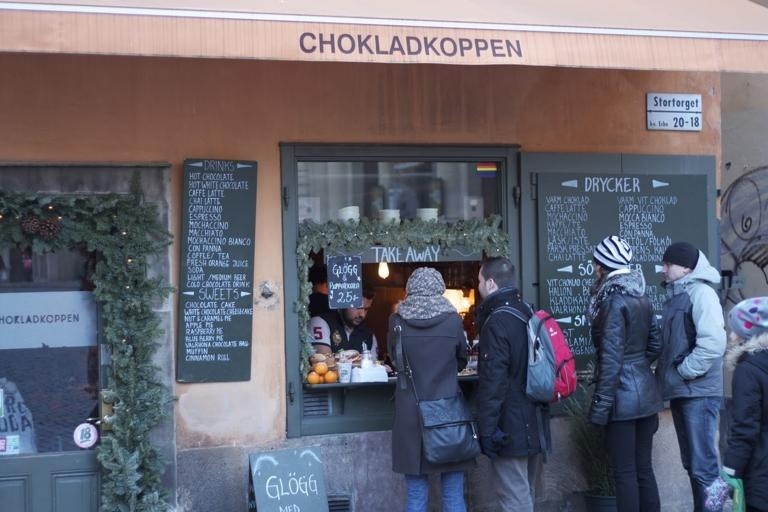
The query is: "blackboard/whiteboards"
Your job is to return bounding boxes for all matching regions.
[326,255,363,309]
[536,172,710,417]
[249,446,329,512]
[176,158,258,383]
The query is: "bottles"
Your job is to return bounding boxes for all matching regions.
[361,351,373,370]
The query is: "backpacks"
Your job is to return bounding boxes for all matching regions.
[482,302,577,403]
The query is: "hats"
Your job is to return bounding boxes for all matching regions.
[406,266,446,295]
[663,242,698,269]
[592,234,633,271]
[726,296,768,339]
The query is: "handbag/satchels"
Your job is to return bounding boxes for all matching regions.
[418,397,481,465]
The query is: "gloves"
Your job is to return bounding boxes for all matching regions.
[704,476,732,512]
[480,430,512,458]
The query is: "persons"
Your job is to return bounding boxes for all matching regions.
[387,266,477,511]
[703,296,767,512]
[309,279,392,373]
[587,235,664,511]
[656,241,727,512]
[473,257,550,511]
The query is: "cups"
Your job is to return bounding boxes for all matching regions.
[337,205,360,226]
[337,362,353,383]
[377,209,402,227]
[416,208,439,224]
